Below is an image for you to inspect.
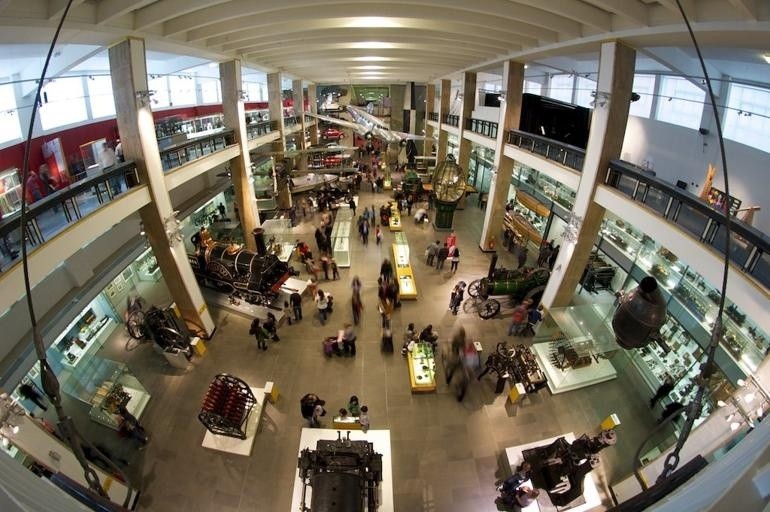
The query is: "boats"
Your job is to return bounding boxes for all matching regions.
[290,173,338,197]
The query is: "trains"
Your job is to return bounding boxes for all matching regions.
[174,220,303,311]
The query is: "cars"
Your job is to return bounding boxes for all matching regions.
[322,143,349,156]
[320,127,346,142]
[323,155,347,168]
[321,114,349,126]
[319,100,347,112]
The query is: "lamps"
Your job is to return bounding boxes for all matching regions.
[736,373,770,419]
[717,395,756,433]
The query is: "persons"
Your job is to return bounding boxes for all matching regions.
[341,323,358,357]
[424,233,460,274]
[651,378,684,425]
[453,327,468,361]
[97,138,130,195]
[282,287,333,326]
[23,163,60,213]
[197,203,226,250]
[299,392,369,430]
[352,258,401,329]
[499,199,560,336]
[134,296,143,310]
[249,312,280,351]
[500,438,592,511]
[450,281,466,316]
[265,139,430,281]
[402,323,438,360]
[29,413,65,442]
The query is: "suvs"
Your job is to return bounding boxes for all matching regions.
[468,253,552,322]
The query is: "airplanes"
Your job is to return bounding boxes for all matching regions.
[300,101,437,153]
